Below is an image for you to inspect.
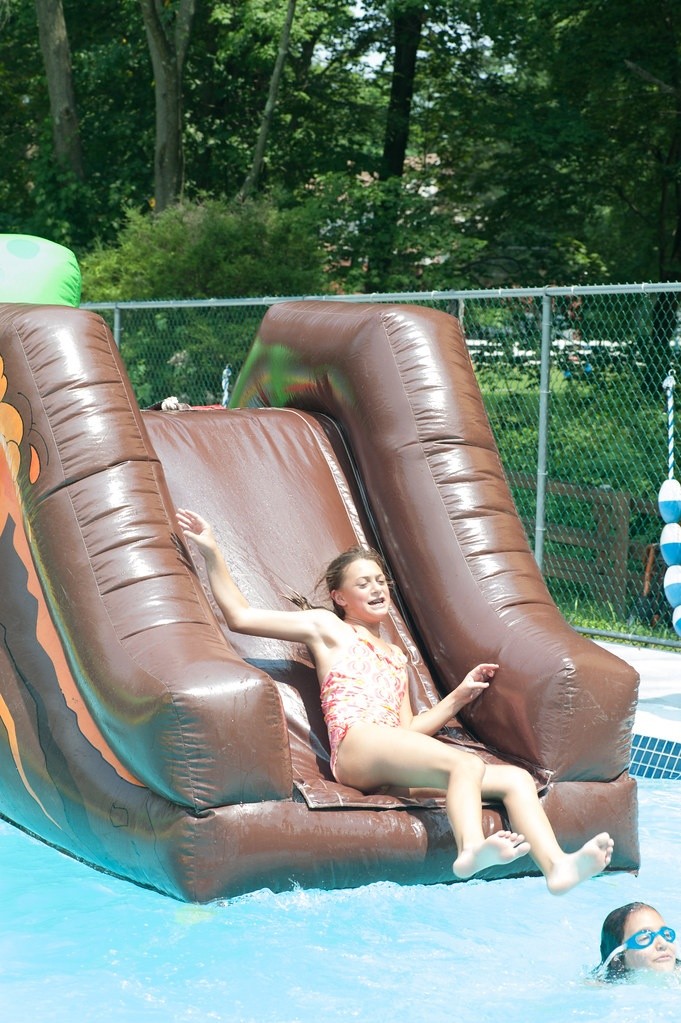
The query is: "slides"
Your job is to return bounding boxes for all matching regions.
[0,299,644,905]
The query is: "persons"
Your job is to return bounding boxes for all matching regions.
[601,902,675,969]
[174,509,615,896]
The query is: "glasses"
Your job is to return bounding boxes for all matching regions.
[602,926,676,968]
